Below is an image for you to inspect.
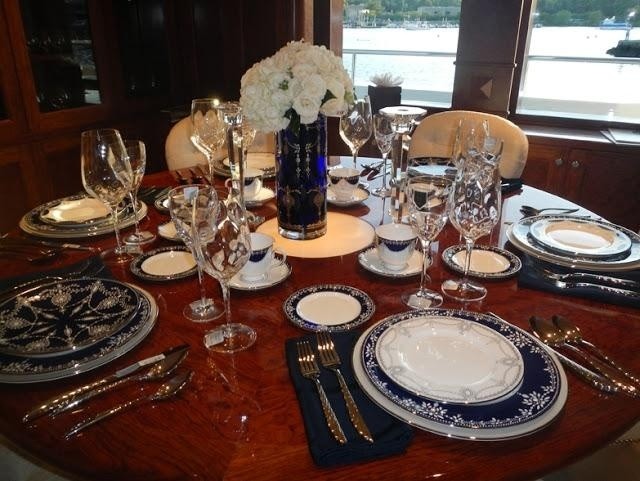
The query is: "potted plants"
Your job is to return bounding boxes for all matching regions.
[368,74,402,117]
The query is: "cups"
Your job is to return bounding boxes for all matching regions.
[326,167,359,200]
[373,222,417,270]
[240,233,286,280]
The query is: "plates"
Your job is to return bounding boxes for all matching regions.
[325,186,371,208]
[357,245,436,277]
[282,284,375,333]
[212,151,276,180]
[19,193,149,242]
[0,272,161,385]
[131,245,198,283]
[229,260,293,292]
[439,245,523,278]
[351,307,568,443]
[505,209,640,272]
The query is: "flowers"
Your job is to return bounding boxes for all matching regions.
[240,38,356,134]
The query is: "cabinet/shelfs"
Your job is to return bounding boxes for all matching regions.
[523,134,640,234]
[0,0,174,229]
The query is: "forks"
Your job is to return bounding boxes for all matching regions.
[541,266,639,298]
[0,237,104,264]
[294,328,376,448]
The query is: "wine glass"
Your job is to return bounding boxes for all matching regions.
[407,113,506,312]
[336,90,394,202]
[79,127,157,267]
[208,351,264,445]
[154,182,258,356]
[188,97,257,188]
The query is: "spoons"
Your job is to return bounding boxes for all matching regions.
[22,339,193,442]
[176,170,188,182]
[527,311,639,403]
[520,204,579,218]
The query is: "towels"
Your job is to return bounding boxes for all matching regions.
[407,158,521,194]
[0,255,117,293]
[283,331,415,470]
[76,184,173,204]
[518,249,640,309]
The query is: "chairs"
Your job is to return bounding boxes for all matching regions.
[406,110,530,181]
[166,115,276,172]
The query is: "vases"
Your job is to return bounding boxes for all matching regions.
[276,109,328,240]
